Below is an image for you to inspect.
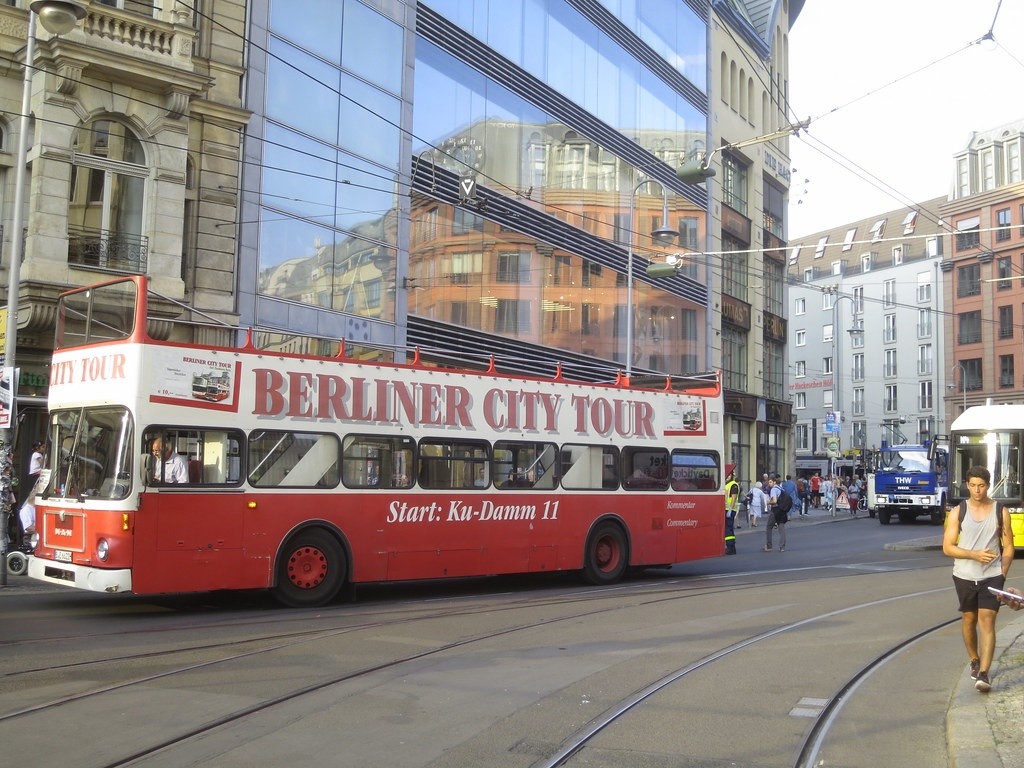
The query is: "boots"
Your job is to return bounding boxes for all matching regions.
[725,541,737,554]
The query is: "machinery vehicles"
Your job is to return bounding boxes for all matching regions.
[874,436,948,526]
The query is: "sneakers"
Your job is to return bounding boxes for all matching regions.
[975,672,990,692]
[969,660,981,680]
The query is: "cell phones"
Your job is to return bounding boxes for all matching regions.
[988,586,1024,603]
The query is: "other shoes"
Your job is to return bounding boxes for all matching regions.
[762,544,773,552]
[779,545,785,552]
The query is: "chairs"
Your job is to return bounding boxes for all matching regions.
[189,459,202,483]
[420,460,668,493]
[138,451,152,486]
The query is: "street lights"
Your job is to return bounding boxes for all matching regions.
[625,179,680,377]
[946,364,966,412]
[1,0,90,585]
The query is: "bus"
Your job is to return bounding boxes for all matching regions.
[26,277,726,609]
[927,403,1024,551]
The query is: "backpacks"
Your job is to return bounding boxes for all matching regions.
[775,486,793,512]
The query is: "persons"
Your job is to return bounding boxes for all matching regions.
[760,477,787,553]
[20,493,37,548]
[475,468,485,487]
[809,472,868,517]
[996,587,1024,611]
[942,464,1016,693]
[746,481,766,526]
[725,471,741,555]
[151,436,190,484]
[761,474,773,513]
[782,475,798,520]
[794,473,811,516]
[28,440,45,481]
[774,473,784,487]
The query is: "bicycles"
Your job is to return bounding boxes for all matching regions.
[856,493,869,512]
